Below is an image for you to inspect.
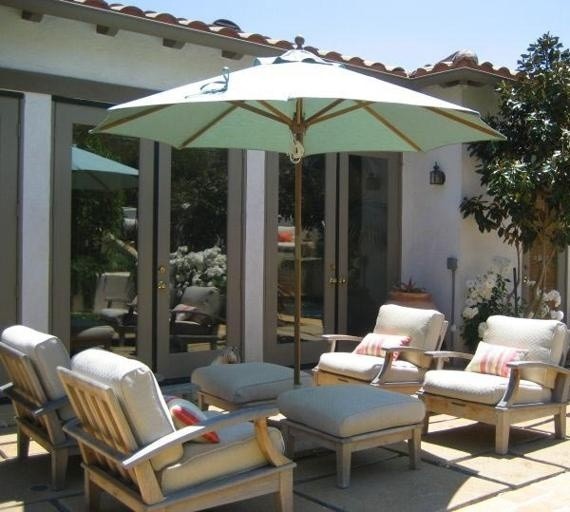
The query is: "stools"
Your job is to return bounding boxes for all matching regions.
[190,362,427,487]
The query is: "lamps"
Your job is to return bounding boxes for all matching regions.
[429,161,445,185]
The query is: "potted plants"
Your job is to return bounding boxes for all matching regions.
[387,276,431,302]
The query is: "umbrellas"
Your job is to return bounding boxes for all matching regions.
[72,144,140,191]
[88,34,509,386]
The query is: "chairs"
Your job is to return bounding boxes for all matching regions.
[1,321,88,490]
[312,302,570,456]
[70,285,222,353]
[55,344,298,510]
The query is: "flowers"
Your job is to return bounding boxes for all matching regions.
[167,244,227,298]
[449,263,565,354]
